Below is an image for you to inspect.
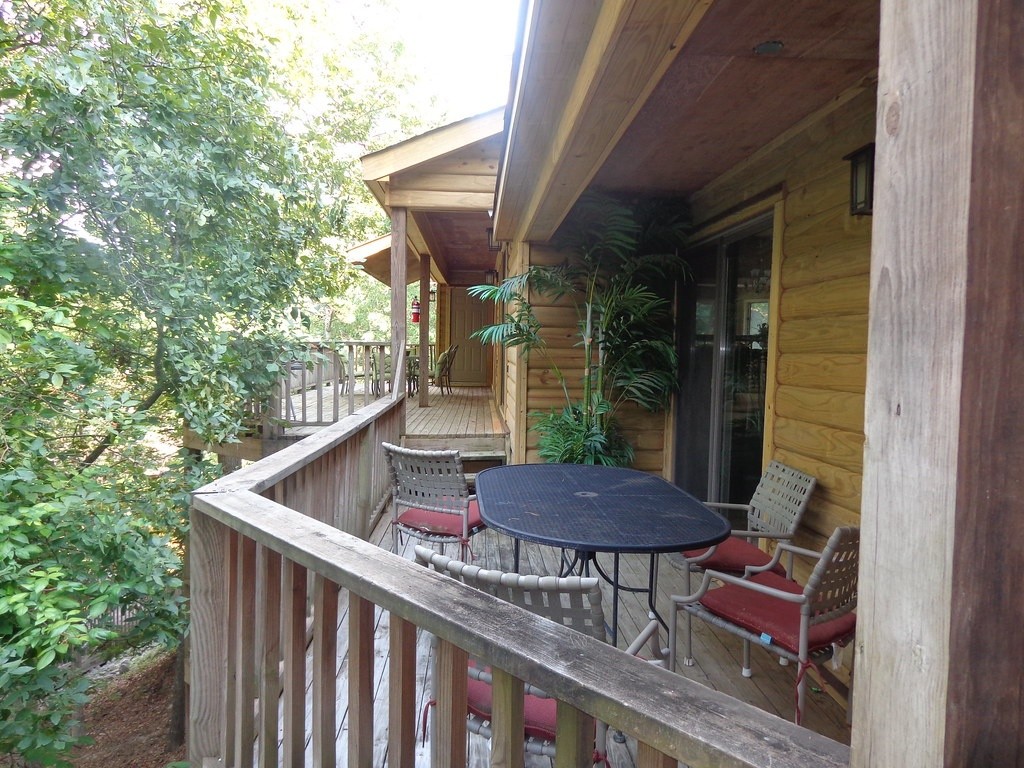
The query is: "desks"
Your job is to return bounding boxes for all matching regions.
[405,355,430,392]
[475,462,731,647]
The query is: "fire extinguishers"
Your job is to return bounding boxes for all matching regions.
[410,295,420,323]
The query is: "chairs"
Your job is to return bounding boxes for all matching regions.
[382,444,488,564]
[370,344,459,398]
[665,526,860,730]
[338,356,357,396]
[664,461,819,666]
[411,545,670,768]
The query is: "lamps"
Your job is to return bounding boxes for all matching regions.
[842,143,875,215]
[429,290,436,302]
[484,270,498,285]
[486,226,503,251]
